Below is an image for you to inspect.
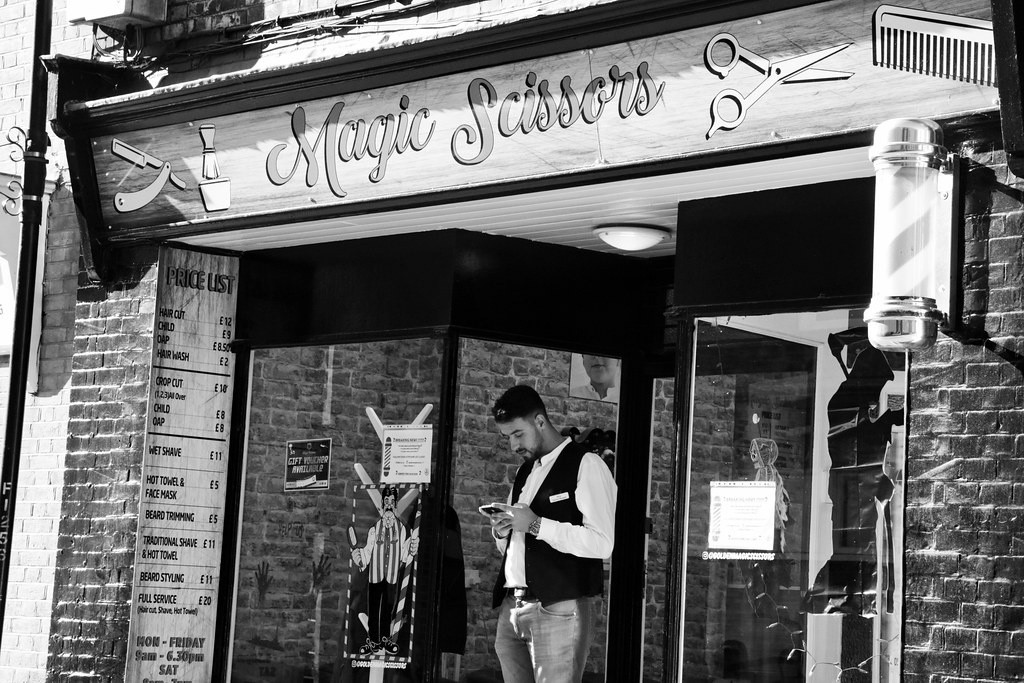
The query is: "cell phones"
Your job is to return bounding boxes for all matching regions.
[481,504,504,515]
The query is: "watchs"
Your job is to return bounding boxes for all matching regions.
[529,516,542,536]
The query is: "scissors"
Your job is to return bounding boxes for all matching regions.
[703,27,853,142]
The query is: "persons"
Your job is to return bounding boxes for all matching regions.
[351,488,421,658]
[478,384,618,683]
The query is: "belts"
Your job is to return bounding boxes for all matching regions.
[502,587,535,597]
